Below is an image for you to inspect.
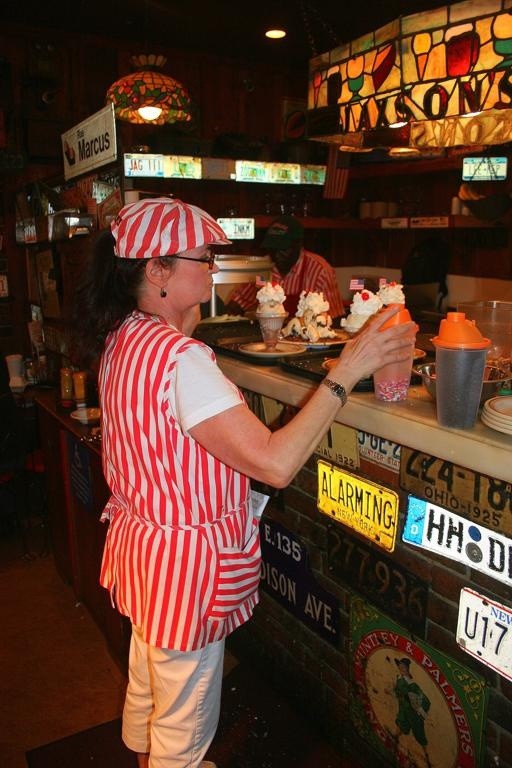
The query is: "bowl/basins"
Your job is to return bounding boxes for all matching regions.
[411,362,511,402]
[71,408,102,423]
[455,299,512,361]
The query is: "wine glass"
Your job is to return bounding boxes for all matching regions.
[255,312,288,351]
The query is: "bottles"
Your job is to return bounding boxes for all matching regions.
[60,367,90,400]
[38,356,49,379]
[25,358,40,385]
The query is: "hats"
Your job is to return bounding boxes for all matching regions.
[259,216,304,250]
[111,196,233,259]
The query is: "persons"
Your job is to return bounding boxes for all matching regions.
[225,214,346,329]
[383,656,432,768]
[76,197,418,768]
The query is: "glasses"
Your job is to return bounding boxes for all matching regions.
[168,253,215,269]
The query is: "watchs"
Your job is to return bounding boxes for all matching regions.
[321,377,347,406]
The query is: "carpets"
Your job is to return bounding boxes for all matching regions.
[23,661,410,768]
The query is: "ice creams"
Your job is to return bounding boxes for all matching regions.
[254,279,290,351]
[377,280,407,308]
[294,287,338,343]
[343,286,384,336]
[410,30,432,82]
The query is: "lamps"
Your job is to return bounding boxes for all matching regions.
[104,1,195,126]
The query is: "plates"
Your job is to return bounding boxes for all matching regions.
[481,395,511,436]
[323,357,363,383]
[277,328,355,348]
[239,342,306,357]
[411,348,427,361]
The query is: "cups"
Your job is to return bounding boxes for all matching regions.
[435,348,487,427]
[371,330,409,401]
[6,354,22,377]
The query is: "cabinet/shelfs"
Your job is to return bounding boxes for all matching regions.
[28,388,154,676]
